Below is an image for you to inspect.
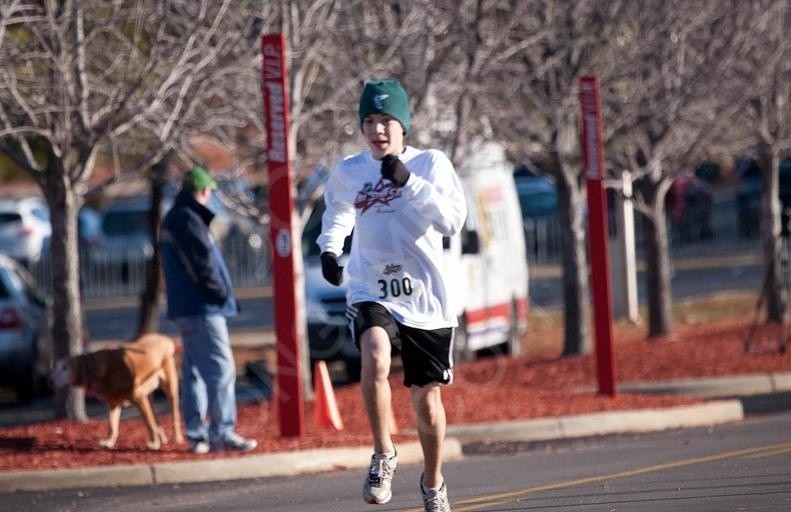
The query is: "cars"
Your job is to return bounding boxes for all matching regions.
[0,254,51,401]
[666,157,791,239]
[0,197,52,269]
[81,176,278,293]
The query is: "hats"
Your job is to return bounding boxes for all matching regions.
[183,167,218,190]
[359,78,411,135]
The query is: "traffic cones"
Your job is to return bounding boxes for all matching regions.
[313,358,345,431]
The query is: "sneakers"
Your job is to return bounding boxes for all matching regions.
[419,471,450,512]
[192,440,209,454]
[363,444,398,504]
[209,434,257,454]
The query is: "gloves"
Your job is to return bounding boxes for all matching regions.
[378,154,410,188]
[322,252,345,285]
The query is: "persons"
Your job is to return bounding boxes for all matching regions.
[316,79,468,512]
[159,166,258,456]
[672,164,712,244]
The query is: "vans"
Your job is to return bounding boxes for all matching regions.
[297,142,530,383]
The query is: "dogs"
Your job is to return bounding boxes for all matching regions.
[48,332,184,451]
